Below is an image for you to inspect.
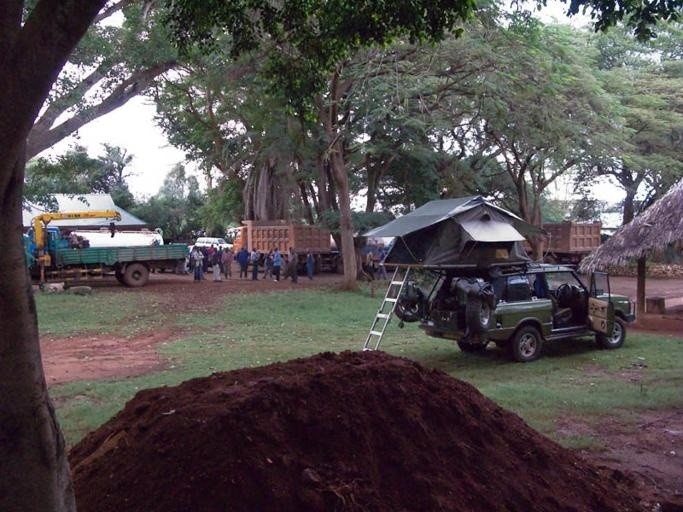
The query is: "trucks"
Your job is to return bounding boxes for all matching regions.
[231,219,330,276]
[21,209,189,287]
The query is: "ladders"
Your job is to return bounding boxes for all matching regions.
[362,265,411,352]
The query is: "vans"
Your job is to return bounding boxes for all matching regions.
[196,237,233,253]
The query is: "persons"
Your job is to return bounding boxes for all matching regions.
[376,246,390,280]
[186,241,300,284]
[364,248,375,280]
[304,246,315,279]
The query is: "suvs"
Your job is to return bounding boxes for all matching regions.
[392,258,637,364]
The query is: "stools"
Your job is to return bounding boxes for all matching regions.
[646,297,666,314]
